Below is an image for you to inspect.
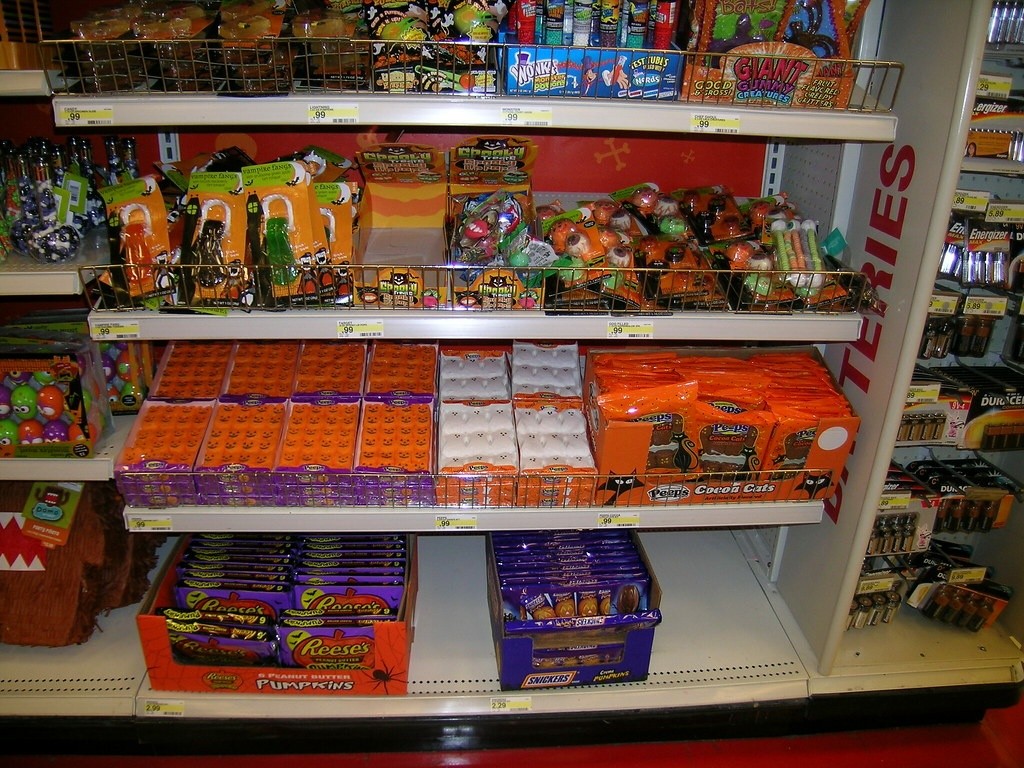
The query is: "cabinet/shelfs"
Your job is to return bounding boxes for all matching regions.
[54,98,897,756]
[0,70,157,757]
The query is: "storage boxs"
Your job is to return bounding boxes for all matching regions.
[135,532,418,697]
[485,528,662,691]
